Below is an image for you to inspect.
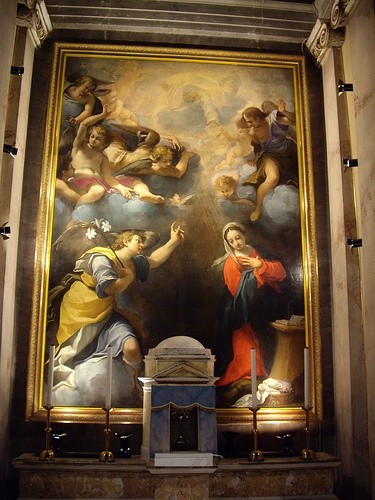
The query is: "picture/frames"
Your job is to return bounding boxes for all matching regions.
[24,41,323,424]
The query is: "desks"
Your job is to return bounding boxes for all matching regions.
[13,451,341,500]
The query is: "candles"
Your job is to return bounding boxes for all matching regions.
[46,344,56,406]
[251,347,257,410]
[104,345,112,409]
[303,347,311,409]
